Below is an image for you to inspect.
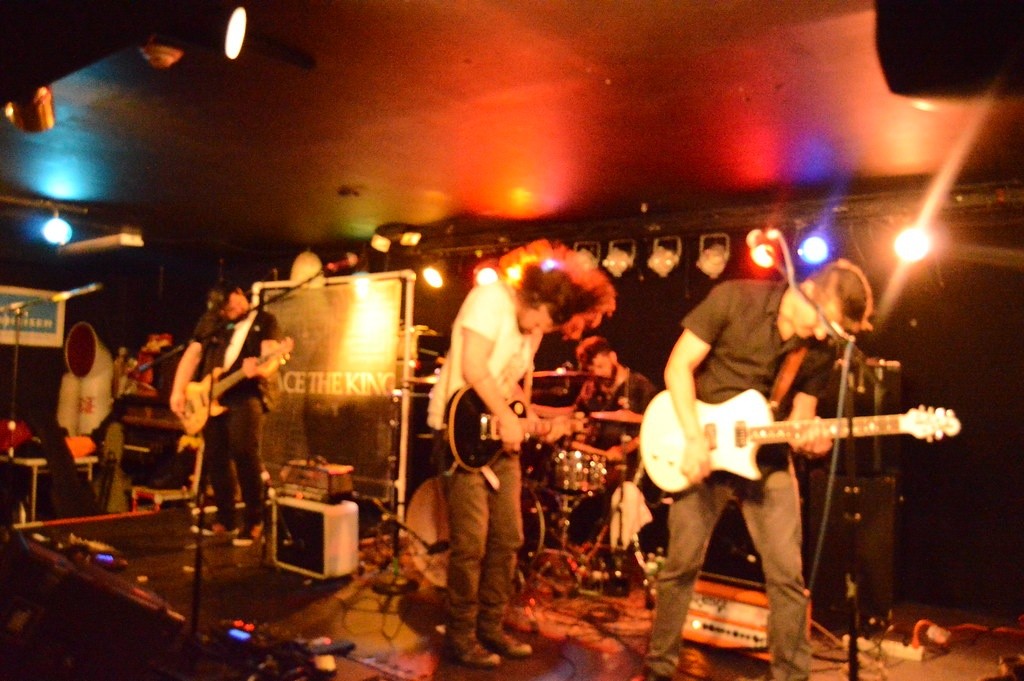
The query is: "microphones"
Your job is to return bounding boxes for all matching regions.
[746,227,780,248]
[327,253,358,270]
[428,540,450,555]
[49,284,102,303]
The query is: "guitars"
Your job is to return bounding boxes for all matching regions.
[637,385,964,490]
[444,385,599,474]
[173,337,295,435]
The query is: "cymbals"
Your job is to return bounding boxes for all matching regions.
[590,408,645,425]
[523,372,611,391]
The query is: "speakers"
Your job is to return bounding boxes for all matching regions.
[272,497,361,578]
[701,497,767,590]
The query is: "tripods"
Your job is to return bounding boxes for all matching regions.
[523,421,657,606]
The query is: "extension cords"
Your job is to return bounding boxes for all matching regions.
[842,635,926,661]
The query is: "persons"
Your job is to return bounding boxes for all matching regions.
[574,336,656,463]
[426,246,615,667]
[644,258,873,681]
[170,280,280,545]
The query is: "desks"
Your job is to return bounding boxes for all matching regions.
[0,455,99,524]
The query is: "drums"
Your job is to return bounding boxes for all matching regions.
[407,446,608,591]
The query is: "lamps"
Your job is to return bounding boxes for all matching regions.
[2,82,54,133]
[574,233,735,278]
[423,260,449,289]
[150,5,247,62]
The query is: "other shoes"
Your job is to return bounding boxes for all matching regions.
[441,641,501,669]
[214,522,236,544]
[478,628,532,657]
[233,520,265,548]
